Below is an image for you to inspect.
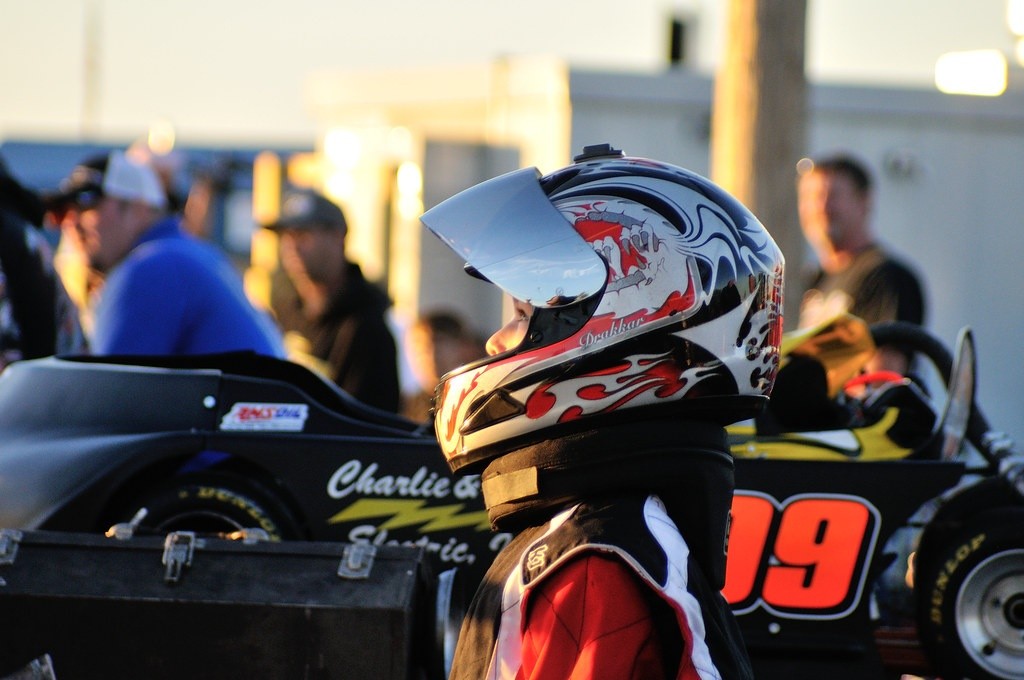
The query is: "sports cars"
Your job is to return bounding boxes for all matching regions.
[0,323,1024,680]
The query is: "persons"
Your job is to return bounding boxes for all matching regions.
[791,148,928,384]
[422,138,787,678]
[264,189,400,416]
[43,154,285,364]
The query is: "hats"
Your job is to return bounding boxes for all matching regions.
[256,186,347,234]
[54,146,170,211]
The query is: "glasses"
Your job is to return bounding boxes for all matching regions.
[46,184,102,222]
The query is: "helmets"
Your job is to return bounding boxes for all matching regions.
[419,141,784,481]
